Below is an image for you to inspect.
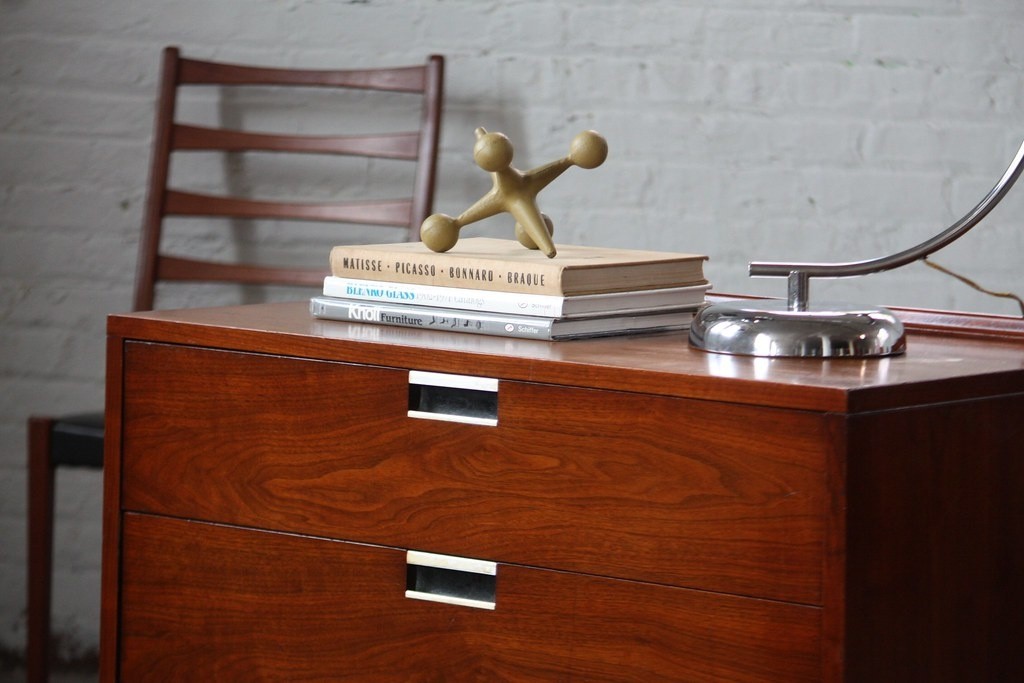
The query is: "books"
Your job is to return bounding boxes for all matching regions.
[308,236,713,342]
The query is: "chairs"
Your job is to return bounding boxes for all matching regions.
[27,48,446,683]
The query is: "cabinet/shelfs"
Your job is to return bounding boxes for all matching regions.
[100,301,1023,683]
